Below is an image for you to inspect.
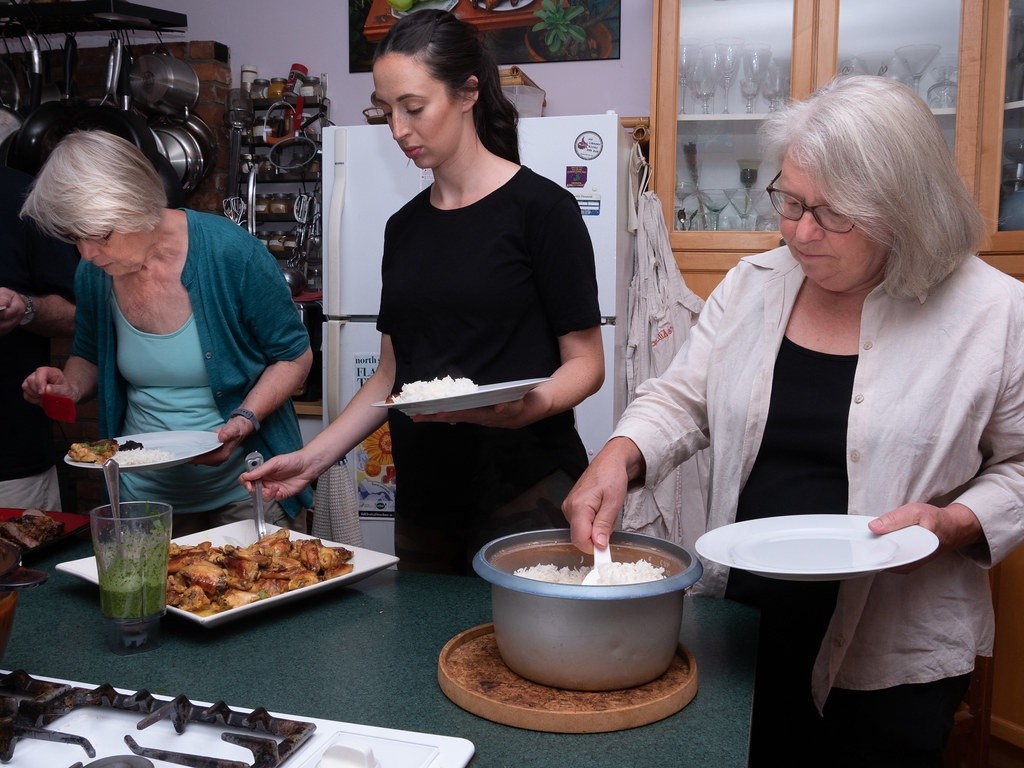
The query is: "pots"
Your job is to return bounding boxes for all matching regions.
[0,30,216,201]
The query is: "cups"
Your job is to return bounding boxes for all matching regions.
[88,500,173,654]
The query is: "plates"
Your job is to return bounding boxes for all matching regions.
[64,430,225,472]
[370,377,556,415]
[390,0,458,19]
[470,0,535,11]
[55,517,400,626]
[695,514,940,580]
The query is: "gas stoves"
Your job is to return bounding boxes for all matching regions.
[0,668,475,767]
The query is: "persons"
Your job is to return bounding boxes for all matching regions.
[22,130,313,539]
[239,9,605,577]
[561,74,1024,768]
[0,166,77,512]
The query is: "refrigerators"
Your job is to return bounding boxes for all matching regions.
[322,109,639,563]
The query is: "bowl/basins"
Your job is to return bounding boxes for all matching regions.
[503,85,545,118]
[473,529,703,691]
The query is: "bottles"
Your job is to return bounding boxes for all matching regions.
[299,76,321,98]
[237,149,322,184]
[268,78,287,98]
[282,63,308,96]
[270,192,292,213]
[249,79,270,99]
[258,229,298,253]
[242,112,313,147]
[255,193,272,212]
[288,192,301,212]
[240,64,258,98]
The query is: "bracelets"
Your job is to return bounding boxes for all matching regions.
[230,408,261,433]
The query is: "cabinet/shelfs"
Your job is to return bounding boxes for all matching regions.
[228,94,331,402]
[648,0,1023,747]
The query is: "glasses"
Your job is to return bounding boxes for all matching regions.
[66,230,113,247]
[765,169,855,233]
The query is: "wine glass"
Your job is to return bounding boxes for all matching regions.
[675,155,778,231]
[678,42,789,113]
[839,44,956,111]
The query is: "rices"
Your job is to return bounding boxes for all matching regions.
[511,555,666,586]
[391,375,478,404]
[103,448,173,466]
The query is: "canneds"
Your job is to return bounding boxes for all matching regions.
[240,63,325,257]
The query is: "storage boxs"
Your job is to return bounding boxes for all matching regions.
[500,85,545,118]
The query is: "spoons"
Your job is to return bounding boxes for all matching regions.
[581,531,613,586]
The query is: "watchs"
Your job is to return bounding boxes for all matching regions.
[19,293,35,326]
